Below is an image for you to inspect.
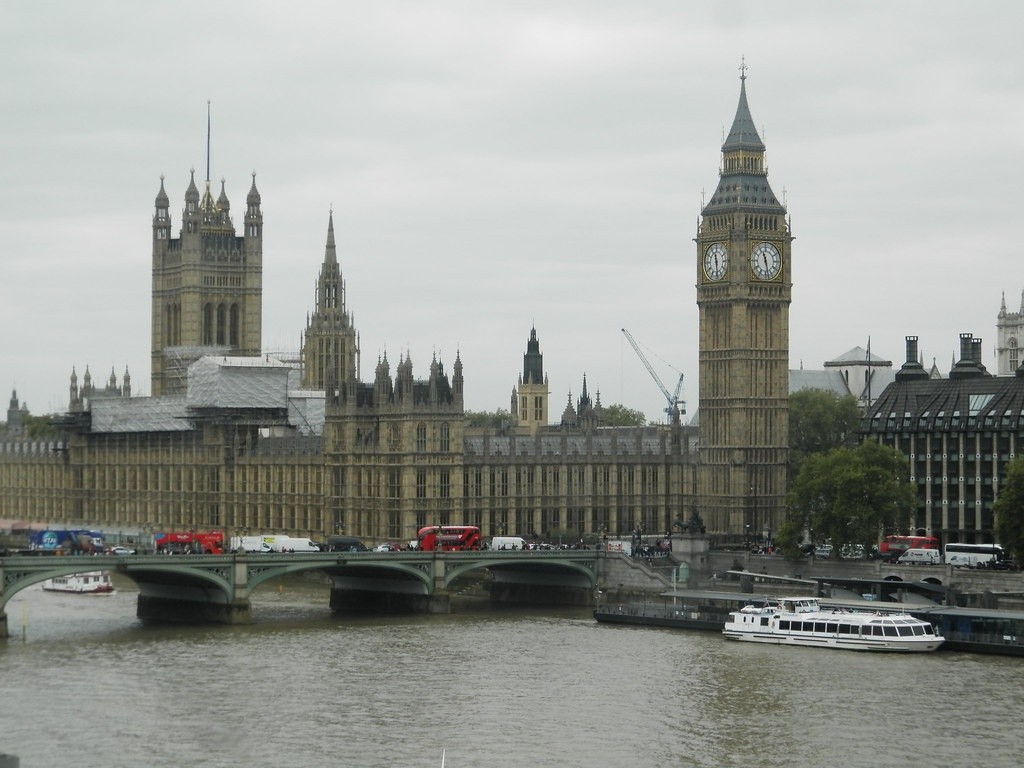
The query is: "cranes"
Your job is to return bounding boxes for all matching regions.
[621,327,687,429]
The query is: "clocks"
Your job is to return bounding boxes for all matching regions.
[704,243,728,280]
[751,242,781,280]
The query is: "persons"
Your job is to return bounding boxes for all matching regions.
[793,567,802,579]
[480,528,672,559]
[757,545,772,555]
[316,542,325,552]
[329,544,335,553]
[183,543,193,555]
[87,543,97,556]
[130,548,138,555]
[162,546,173,555]
[759,566,766,574]
[387,542,424,553]
[266,546,294,554]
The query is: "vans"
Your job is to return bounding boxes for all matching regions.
[897,549,941,565]
[839,544,880,559]
[326,536,369,553]
[230,534,321,554]
[492,536,529,550]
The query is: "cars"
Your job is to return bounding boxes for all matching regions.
[110,545,136,555]
[373,544,401,553]
[528,543,570,550]
[805,543,832,559]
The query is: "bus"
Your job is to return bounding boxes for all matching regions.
[152,529,224,556]
[417,526,480,551]
[880,535,940,553]
[27,529,104,555]
[945,542,1006,569]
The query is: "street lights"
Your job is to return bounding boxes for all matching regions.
[745,525,750,550]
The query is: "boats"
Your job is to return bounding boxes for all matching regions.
[593,582,781,633]
[722,597,946,653]
[42,571,114,592]
